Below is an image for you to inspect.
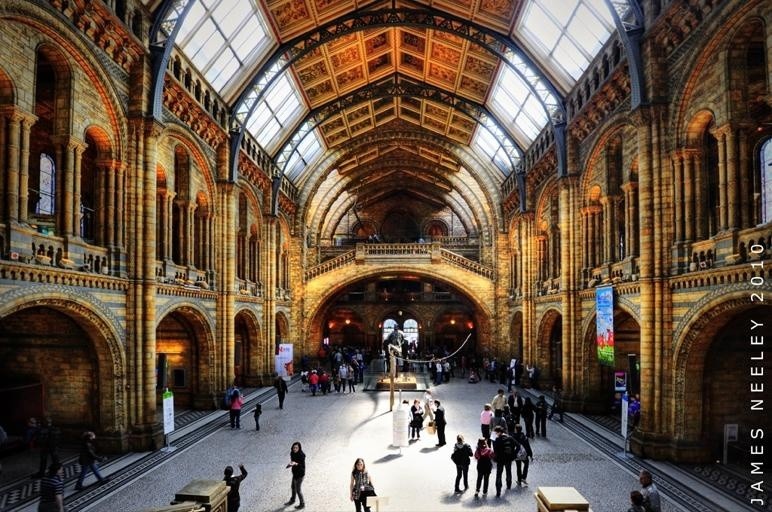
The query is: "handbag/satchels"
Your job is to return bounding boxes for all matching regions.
[477,456,492,472]
[514,444,527,461]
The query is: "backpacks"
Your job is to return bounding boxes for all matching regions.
[498,440,513,463]
[451,451,466,465]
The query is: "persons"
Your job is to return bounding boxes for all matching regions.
[628,491,646,512]
[27,416,68,478]
[74,431,103,489]
[349,458,377,512]
[38,462,65,512]
[274,376,288,409]
[418,236,425,243]
[286,442,306,509]
[226,382,262,431]
[300,343,366,396]
[639,470,661,512]
[368,232,381,243]
[223,464,248,512]
[402,340,564,497]
[388,323,405,348]
